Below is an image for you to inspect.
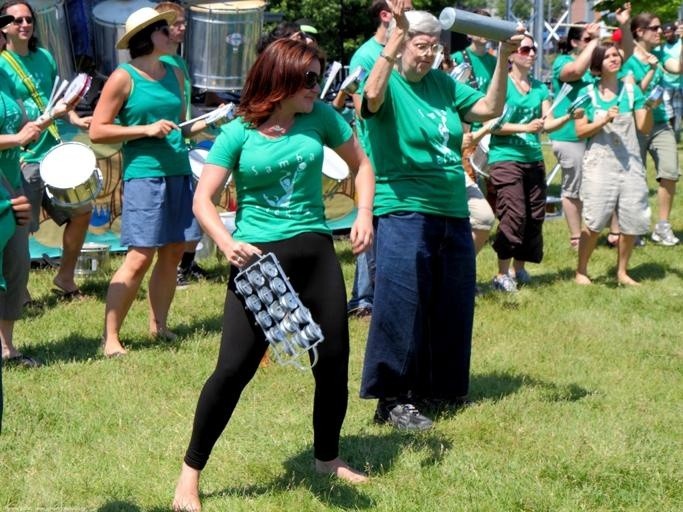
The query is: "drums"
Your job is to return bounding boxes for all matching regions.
[0,0,78,92]
[189,148,232,206]
[38,141,103,209]
[643,85,665,109]
[91,0,159,81]
[450,62,472,82]
[568,95,590,116]
[321,145,355,221]
[205,103,236,129]
[179,0,272,94]
[76,134,124,234]
[63,73,93,105]
[342,66,367,93]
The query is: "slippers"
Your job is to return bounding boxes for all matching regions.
[49,285,91,304]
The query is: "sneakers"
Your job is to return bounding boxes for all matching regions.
[492,267,531,294]
[371,397,434,436]
[650,220,680,248]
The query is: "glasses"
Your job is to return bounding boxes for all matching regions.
[519,45,539,57]
[300,68,326,91]
[583,37,592,44]
[158,23,172,39]
[13,15,35,25]
[413,42,446,59]
[650,24,665,34]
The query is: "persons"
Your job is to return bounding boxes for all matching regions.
[0,0,224,367]
[345,0,682,313]
[172,0,524,512]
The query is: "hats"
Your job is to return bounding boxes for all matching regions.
[113,6,178,52]
